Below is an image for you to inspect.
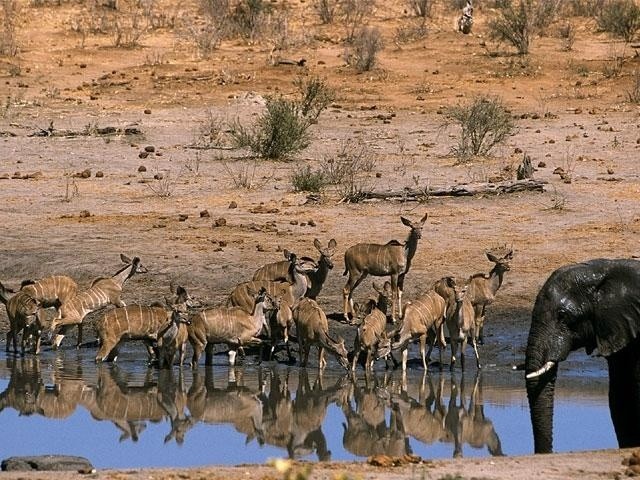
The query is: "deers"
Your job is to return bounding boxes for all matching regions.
[17,275,78,346]
[47,253,148,345]
[98,237,512,370]
[341,211,428,327]
[2,292,38,355]
[0,365,507,466]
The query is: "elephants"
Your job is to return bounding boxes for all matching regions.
[512,258,639,454]
